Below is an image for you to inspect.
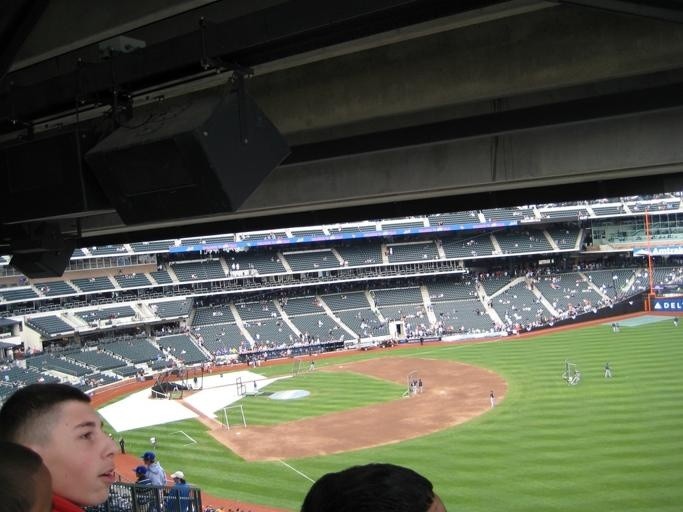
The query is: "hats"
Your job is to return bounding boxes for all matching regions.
[132,466,146,474]
[140,451,154,459]
[170,470,184,478]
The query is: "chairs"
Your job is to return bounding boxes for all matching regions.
[0,186,682,412]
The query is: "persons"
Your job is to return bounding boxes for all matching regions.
[299,463,448,512]
[0,383,117,511]
[0,442,55,512]
[81,433,254,510]
[0,193,682,408]
